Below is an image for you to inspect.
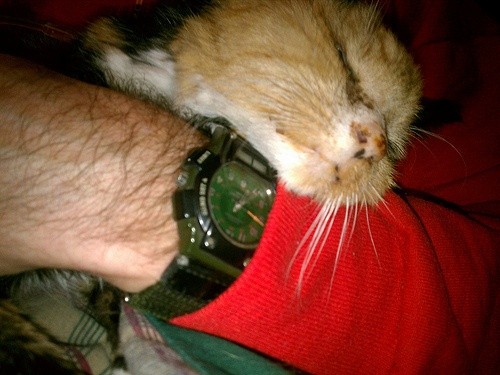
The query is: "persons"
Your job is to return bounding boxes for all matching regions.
[1,2,500,375]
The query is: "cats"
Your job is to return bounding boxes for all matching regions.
[7,0,467,334]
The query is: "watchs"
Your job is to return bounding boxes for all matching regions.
[124,121,276,321]
